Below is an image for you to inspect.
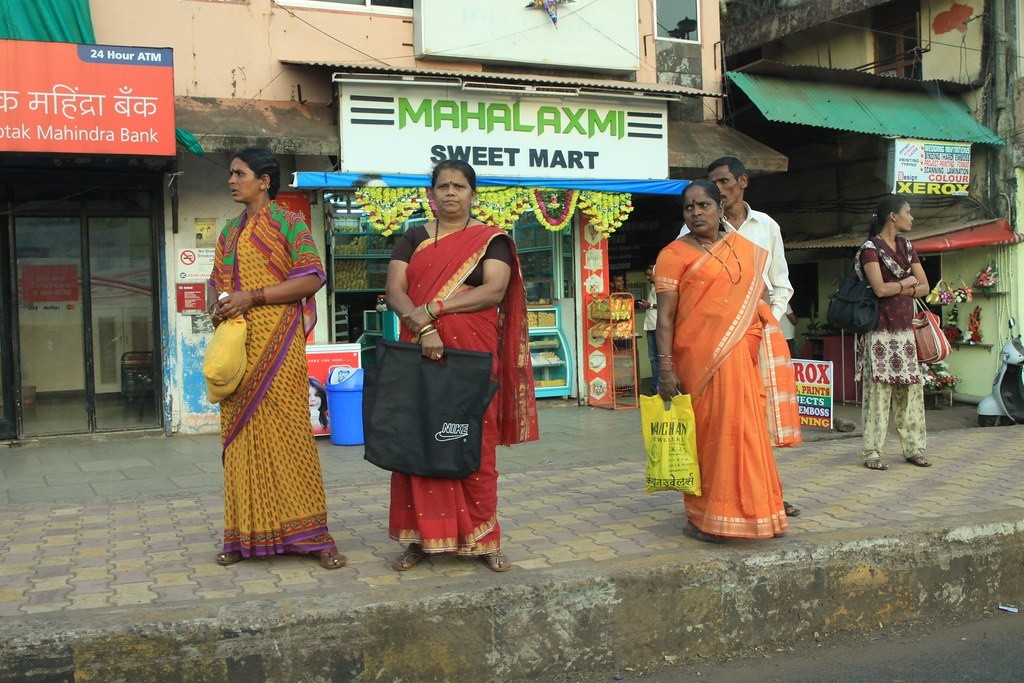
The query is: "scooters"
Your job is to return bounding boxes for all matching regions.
[977,316,1024,427]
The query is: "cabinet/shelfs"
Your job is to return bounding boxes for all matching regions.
[336,214,575,403]
[946,291,1010,351]
[585,292,638,410]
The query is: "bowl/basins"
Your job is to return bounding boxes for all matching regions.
[22,386,37,408]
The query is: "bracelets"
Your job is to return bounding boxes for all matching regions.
[909,285,916,297]
[657,354,676,383]
[419,324,438,336]
[913,276,920,286]
[650,303,654,309]
[897,281,904,294]
[425,300,444,320]
[250,285,267,307]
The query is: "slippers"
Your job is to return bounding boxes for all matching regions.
[783,501,800,517]
[681,520,727,543]
[317,549,345,570]
[906,455,931,467]
[393,551,430,570]
[216,552,242,566]
[863,459,889,470]
[480,552,512,572]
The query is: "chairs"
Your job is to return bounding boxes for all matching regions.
[121,350,157,424]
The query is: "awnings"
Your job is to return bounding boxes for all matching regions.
[723,59,1007,146]
[783,217,1016,251]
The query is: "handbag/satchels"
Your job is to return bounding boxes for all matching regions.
[912,296,952,364]
[640,389,702,497]
[827,238,883,334]
[363,340,500,478]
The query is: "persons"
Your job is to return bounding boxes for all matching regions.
[638,264,661,397]
[653,180,770,544]
[207,145,350,569]
[385,159,512,573]
[676,156,802,518]
[777,303,799,359]
[855,193,930,471]
[609,273,640,397]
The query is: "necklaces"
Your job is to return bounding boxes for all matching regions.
[693,232,742,284]
[879,234,888,243]
[435,217,470,247]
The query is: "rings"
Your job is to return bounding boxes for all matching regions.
[437,353,442,360]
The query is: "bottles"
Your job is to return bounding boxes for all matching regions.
[377,295,386,304]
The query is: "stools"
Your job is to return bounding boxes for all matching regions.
[926,389,953,408]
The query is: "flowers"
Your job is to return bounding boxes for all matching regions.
[920,360,965,392]
[924,259,1001,345]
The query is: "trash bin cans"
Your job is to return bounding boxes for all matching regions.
[324,367,367,447]
